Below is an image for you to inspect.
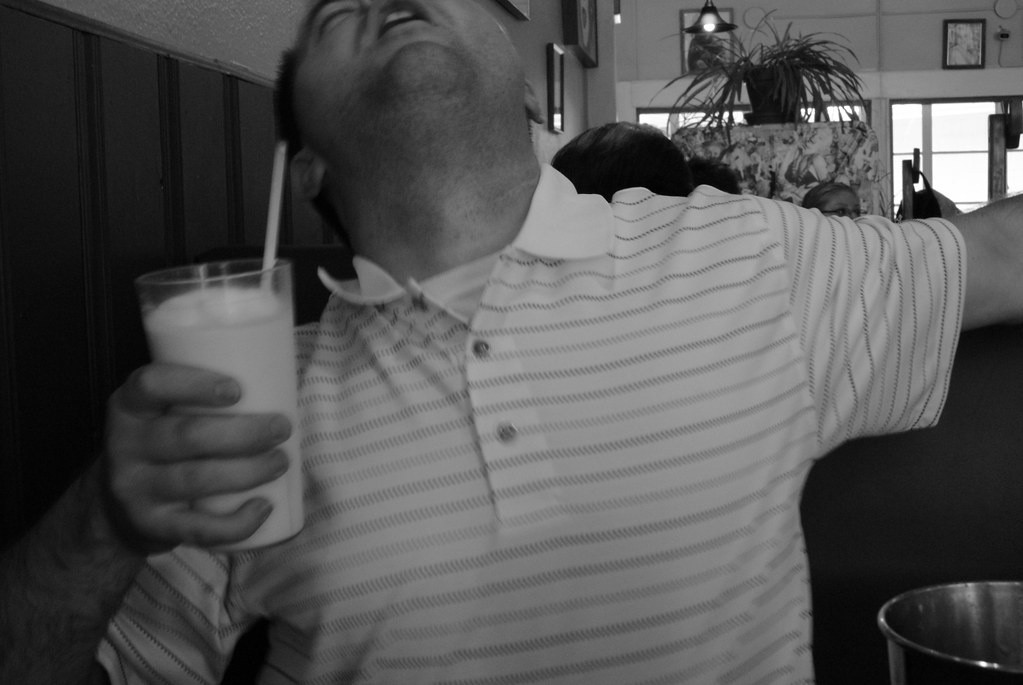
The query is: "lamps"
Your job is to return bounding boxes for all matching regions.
[680,0,738,34]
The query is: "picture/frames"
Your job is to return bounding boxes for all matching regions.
[562,0,598,68]
[942,18,986,69]
[547,43,565,135]
[679,8,735,75]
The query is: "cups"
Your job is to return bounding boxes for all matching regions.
[135,258,304,552]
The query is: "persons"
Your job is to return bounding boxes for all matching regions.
[688,155,740,194]
[108,120,695,549]
[802,182,860,220]
[87,0,1023,685]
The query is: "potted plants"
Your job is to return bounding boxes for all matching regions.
[644,8,875,154]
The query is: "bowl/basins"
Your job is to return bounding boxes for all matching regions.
[878,580,1023,685]
